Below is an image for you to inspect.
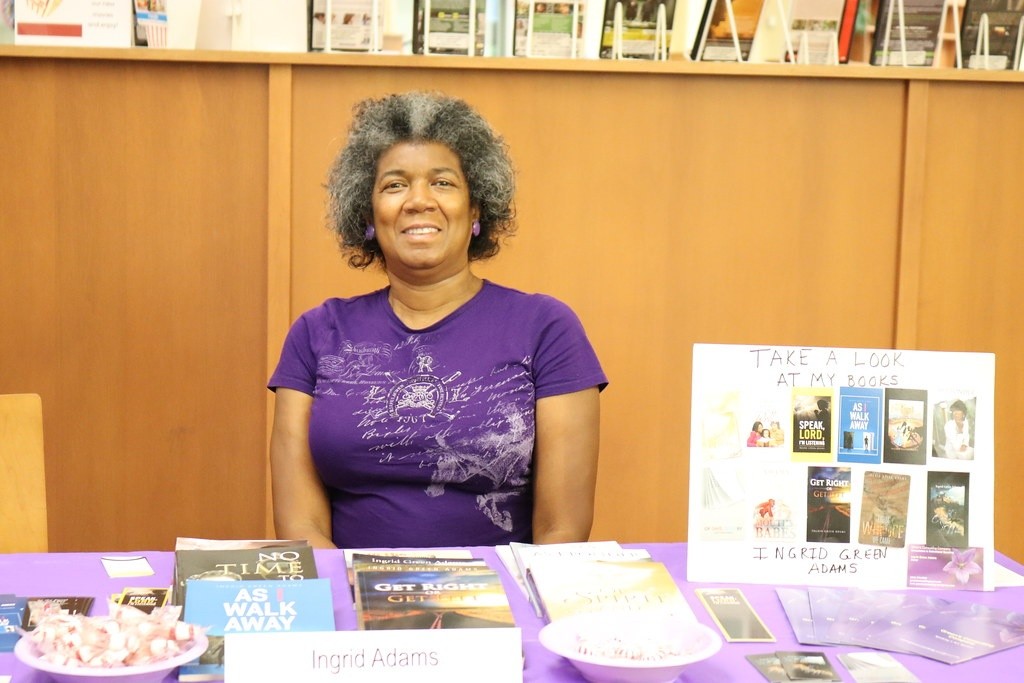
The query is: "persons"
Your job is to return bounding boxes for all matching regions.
[267,93,609,551]
[747,420,785,447]
[943,400,975,459]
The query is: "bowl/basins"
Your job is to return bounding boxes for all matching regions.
[15,616,209,683]
[539,613,722,683]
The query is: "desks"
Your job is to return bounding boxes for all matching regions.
[0,541,1024,683]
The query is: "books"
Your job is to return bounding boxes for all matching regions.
[492,539,697,624]
[175,537,337,682]
[344,550,524,658]
[14,0,1024,71]
